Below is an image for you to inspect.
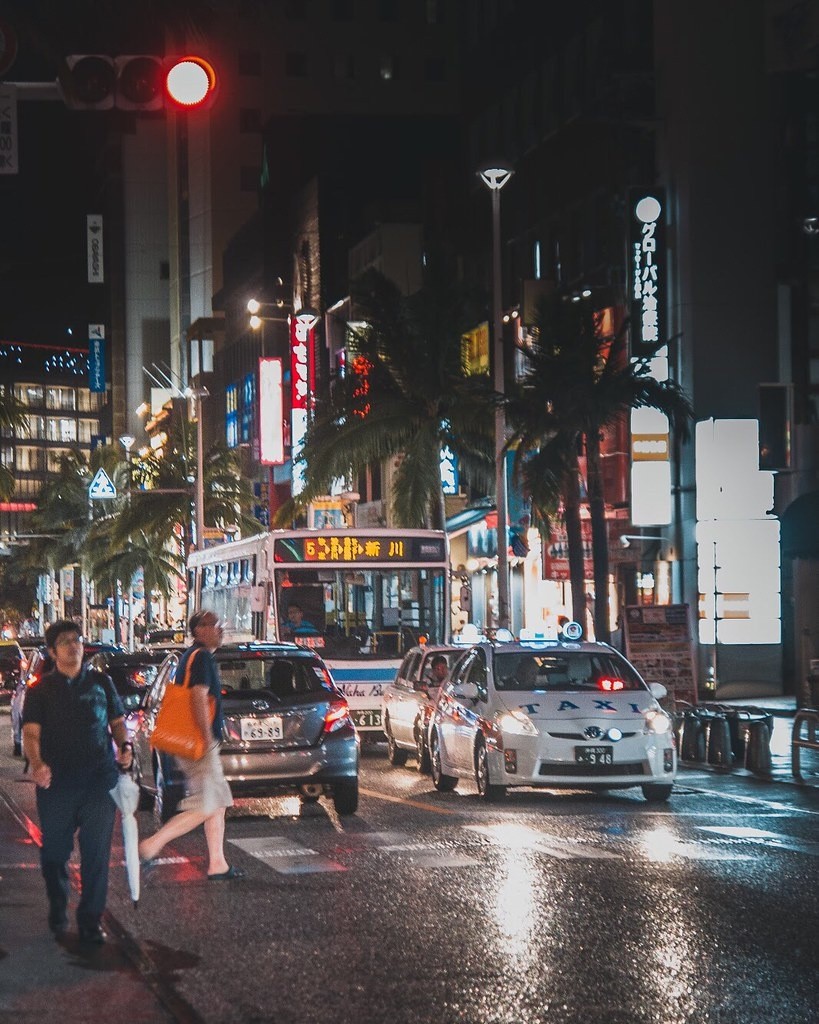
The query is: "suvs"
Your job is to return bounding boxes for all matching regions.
[123,640,361,824]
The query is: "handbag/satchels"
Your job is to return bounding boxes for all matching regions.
[149,648,217,761]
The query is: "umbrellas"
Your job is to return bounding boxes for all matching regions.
[109,740,140,907]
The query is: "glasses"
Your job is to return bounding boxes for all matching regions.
[431,665,449,672]
[54,634,84,648]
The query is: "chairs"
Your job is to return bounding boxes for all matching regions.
[566,656,594,685]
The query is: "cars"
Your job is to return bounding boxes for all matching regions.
[425,638,679,803]
[0,625,185,777]
[381,640,518,772]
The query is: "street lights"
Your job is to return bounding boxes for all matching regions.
[472,155,520,627]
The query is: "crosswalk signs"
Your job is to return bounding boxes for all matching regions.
[88,466,116,498]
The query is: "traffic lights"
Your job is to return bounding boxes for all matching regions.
[68,53,220,109]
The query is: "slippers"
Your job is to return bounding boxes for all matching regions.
[206,865,249,880]
[138,840,160,888]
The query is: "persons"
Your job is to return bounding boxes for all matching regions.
[432,656,448,680]
[139,612,248,887]
[504,658,539,689]
[21,620,133,943]
[280,604,316,633]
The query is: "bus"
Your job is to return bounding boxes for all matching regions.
[185,526,472,743]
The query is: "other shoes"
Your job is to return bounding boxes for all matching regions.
[47,893,71,934]
[75,911,107,944]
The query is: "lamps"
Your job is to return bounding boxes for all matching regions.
[250,316,289,330]
[618,535,673,548]
[274,296,293,308]
[248,299,293,314]
[273,276,301,289]
